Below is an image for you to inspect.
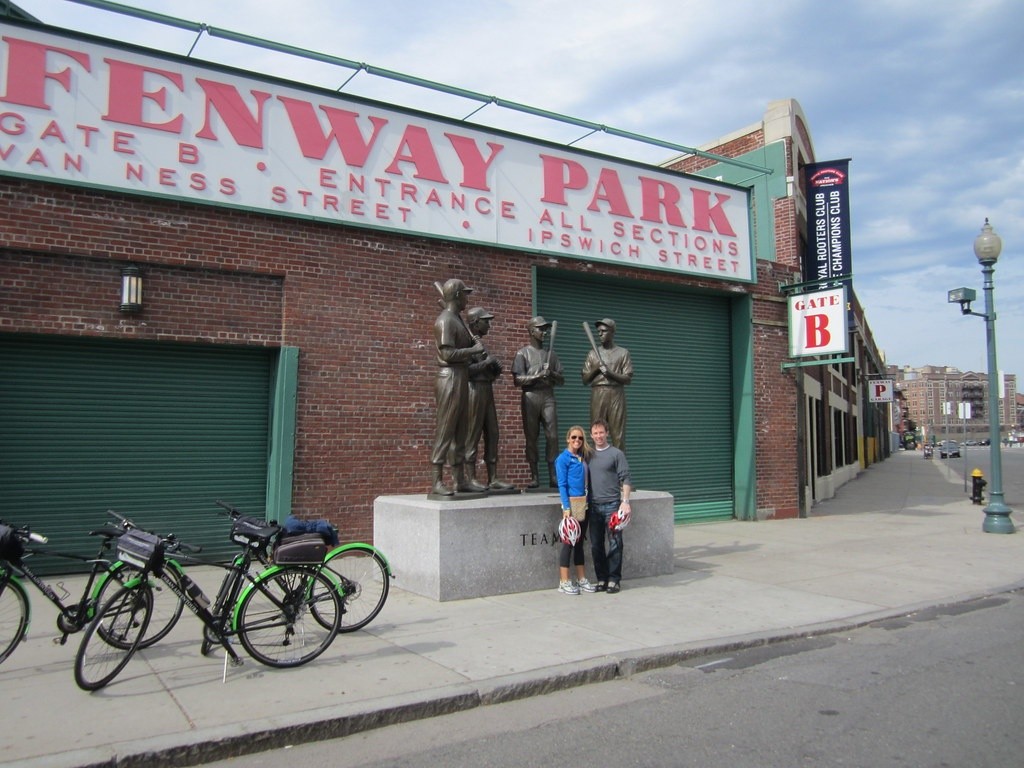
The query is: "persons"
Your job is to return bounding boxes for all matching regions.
[585,418,631,593]
[511,315,565,487]
[430,278,515,495]
[581,318,633,486]
[554,425,599,594]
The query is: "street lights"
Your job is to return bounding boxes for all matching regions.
[947,216,1015,535]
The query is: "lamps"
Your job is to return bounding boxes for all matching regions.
[118,263,144,313]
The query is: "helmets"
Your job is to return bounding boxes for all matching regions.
[559,517,581,547]
[609,512,630,531]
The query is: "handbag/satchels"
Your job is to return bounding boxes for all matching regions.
[568,496,588,521]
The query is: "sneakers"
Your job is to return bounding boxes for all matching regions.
[607,581,620,592]
[558,580,579,594]
[597,581,607,591]
[575,578,596,593]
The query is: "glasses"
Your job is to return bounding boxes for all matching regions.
[570,436,583,440]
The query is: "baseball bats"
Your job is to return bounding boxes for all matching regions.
[433,280,487,355]
[582,321,607,376]
[543,320,558,378]
[437,298,447,309]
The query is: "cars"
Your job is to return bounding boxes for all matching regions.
[960,440,976,447]
[978,438,990,446]
[927,439,956,446]
[939,441,960,459]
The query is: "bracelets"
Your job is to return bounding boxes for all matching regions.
[622,498,629,503]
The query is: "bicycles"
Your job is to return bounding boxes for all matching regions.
[74,510,344,692]
[0,519,185,666]
[200,499,396,658]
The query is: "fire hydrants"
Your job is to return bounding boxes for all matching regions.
[969,468,987,506]
